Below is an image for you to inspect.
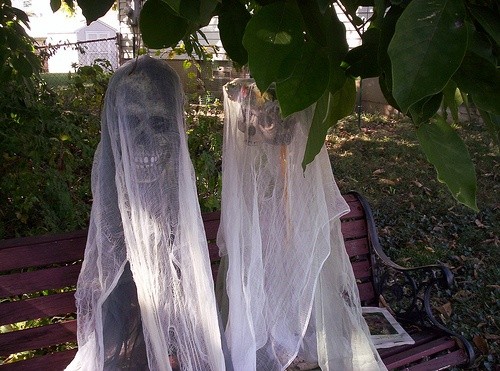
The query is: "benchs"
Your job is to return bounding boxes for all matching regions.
[0,189,475,371]
